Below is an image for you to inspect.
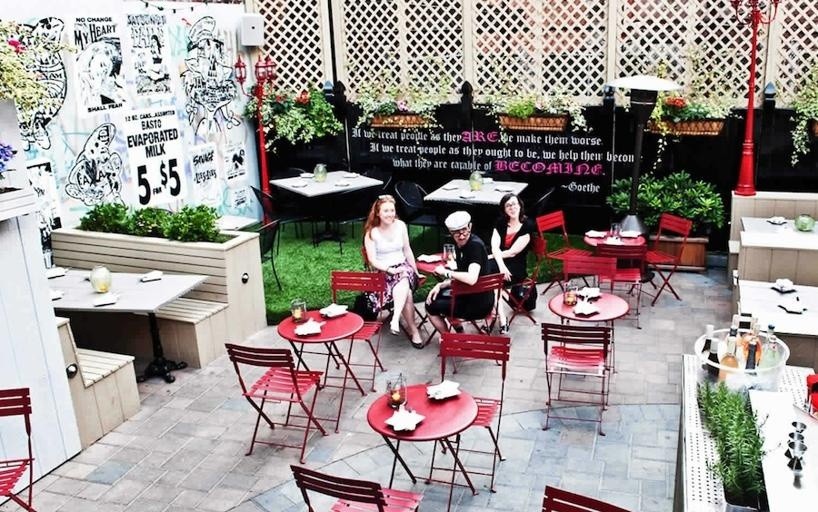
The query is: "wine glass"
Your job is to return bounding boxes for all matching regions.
[784,420,807,472]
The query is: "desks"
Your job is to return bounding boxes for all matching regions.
[676,191,817,512]
[47,267,211,381]
[276,309,368,434]
[368,383,481,511]
[549,289,629,374]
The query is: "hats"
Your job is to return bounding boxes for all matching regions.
[444,210,471,232]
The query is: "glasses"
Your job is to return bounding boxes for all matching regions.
[452,229,471,237]
[378,194,393,200]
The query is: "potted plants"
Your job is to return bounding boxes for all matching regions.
[607,168,724,270]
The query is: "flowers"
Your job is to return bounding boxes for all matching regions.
[645,47,733,119]
[350,70,444,129]
[479,83,593,133]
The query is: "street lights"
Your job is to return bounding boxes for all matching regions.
[730,0,780,197]
[235,51,276,230]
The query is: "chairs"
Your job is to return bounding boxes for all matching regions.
[251,159,392,254]
[226,342,329,464]
[0,388,39,511]
[241,221,282,291]
[539,323,614,436]
[292,466,425,511]
[318,271,387,392]
[424,333,511,493]
[536,210,692,330]
[541,486,627,512]
[396,179,556,251]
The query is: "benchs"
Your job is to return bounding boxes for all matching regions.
[53,311,140,452]
[50,227,268,371]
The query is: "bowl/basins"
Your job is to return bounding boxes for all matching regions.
[694,328,791,393]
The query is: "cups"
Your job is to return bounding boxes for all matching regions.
[385,376,408,409]
[291,298,308,324]
[443,244,454,262]
[611,223,621,238]
[563,283,577,305]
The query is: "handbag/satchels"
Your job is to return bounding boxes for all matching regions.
[352,293,378,321]
[509,285,537,311]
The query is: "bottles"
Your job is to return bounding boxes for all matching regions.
[693,312,782,387]
[90,265,112,293]
[794,214,816,232]
[314,165,328,181]
[469,172,482,190]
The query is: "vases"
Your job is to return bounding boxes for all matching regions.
[370,112,426,128]
[496,113,570,131]
[645,120,725,135]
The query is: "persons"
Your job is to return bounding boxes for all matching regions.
[146,35,165,82]
[479,193,534,335]
[363,194,424,349]
[425,211,495,357]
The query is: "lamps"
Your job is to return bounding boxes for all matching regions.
[235,52,278,229]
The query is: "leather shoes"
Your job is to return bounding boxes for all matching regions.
[390,330,401,336]
[412,340,423,349]
[481,325,491,334]
[499,317,510,334]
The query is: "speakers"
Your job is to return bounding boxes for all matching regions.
[240,13,263,46]
